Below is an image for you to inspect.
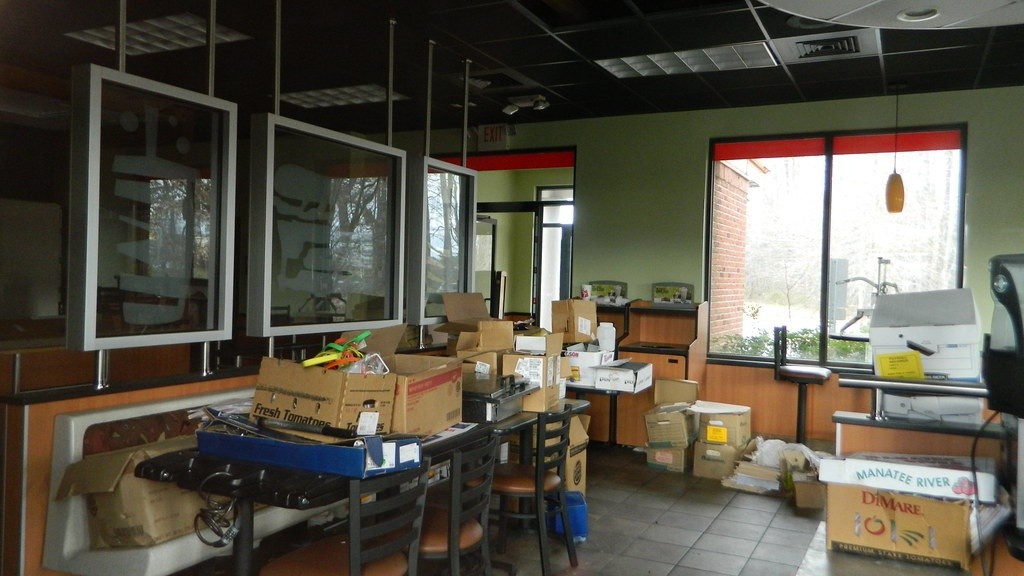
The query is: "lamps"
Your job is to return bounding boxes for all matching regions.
[886,85,905,214]
[502,94,550,115]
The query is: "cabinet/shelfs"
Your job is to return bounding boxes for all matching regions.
[570,296,708,450]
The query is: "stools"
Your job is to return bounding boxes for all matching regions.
[773,325,833,444]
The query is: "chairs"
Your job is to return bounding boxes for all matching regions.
[365,433,501,576]
[462,403,579,576]
[258,456,433,576]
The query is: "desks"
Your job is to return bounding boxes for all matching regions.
[566,386,622,399]
[135,398,592,576]
[830,411,1010,465]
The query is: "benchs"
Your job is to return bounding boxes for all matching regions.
[43,387,350,576]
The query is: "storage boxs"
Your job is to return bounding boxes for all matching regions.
[645,442,694,474]
[644,402,698,448]
[50,294,654,554]
[692,439,756,480]
[868,287,984,424]
[826,482,973,571]
[793,481,826,509]
[819,451,997,502]
[654,377,700,405]
[698,409,751,447]
